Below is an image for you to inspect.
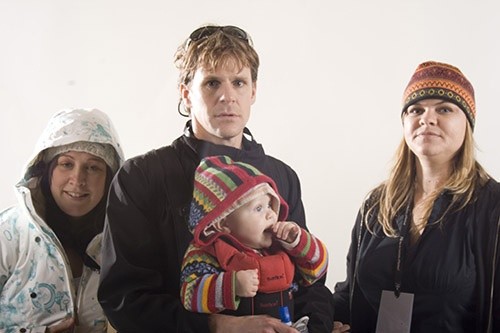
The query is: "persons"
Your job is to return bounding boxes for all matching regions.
[333,61,500,333]
[179,154,330,333]
[96,24,335,333]
[0,108,126,333]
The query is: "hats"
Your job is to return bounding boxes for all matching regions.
[42,142,118,173]
[400,61,476,132]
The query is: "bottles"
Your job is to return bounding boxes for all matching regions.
[279,306,294,327]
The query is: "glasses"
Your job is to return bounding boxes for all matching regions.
[185,26,249,52]
